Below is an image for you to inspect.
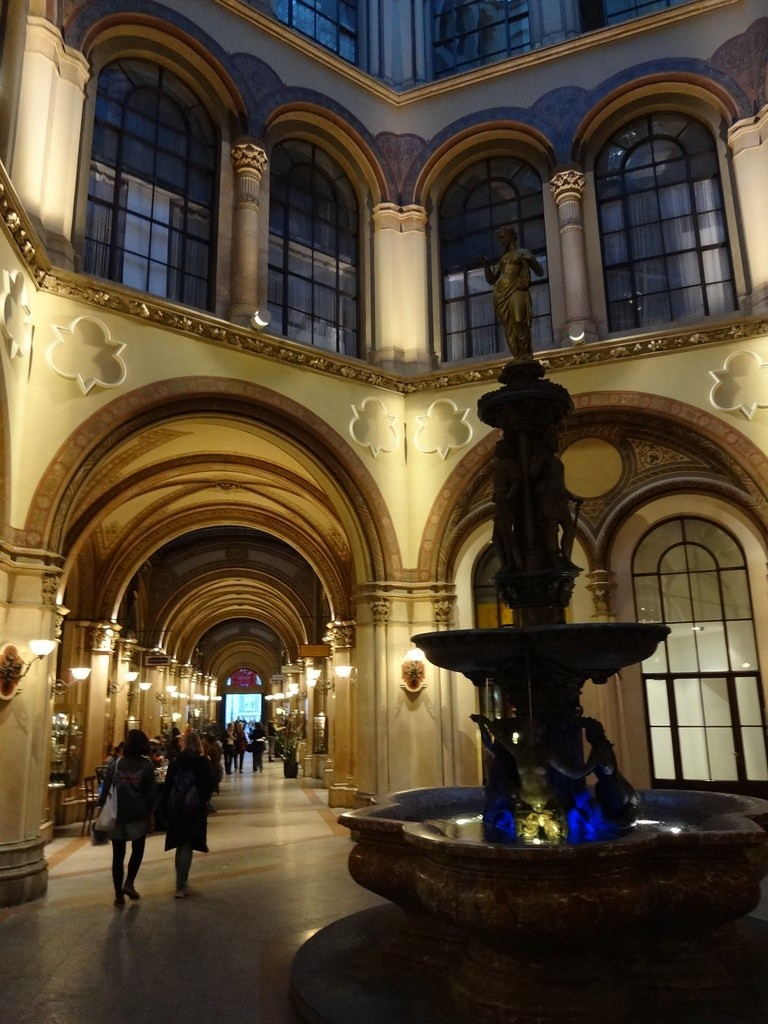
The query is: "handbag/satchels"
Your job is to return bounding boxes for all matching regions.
[166,775,200,822]
[94,783,118,833]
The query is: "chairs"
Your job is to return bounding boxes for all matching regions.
[80,776,101,836]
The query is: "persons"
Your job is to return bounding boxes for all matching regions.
[98,729,154,906]
[478,225,544,366]
[472,713,570,846]
[476,715,520,824]
[224,716,277,775]
[200,721,223,794]
[164,732,213,898]
[542,717,639,827]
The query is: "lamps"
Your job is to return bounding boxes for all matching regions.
[568,324,586,347]
[0,639,178,704]
[251,309,271,330]
[289,662,357,699]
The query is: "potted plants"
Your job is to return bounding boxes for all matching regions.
[282,735,299,778]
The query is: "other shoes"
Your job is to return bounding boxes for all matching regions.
[123,887,141,901]
[173,884,193,898]
[113,897,125,909]
